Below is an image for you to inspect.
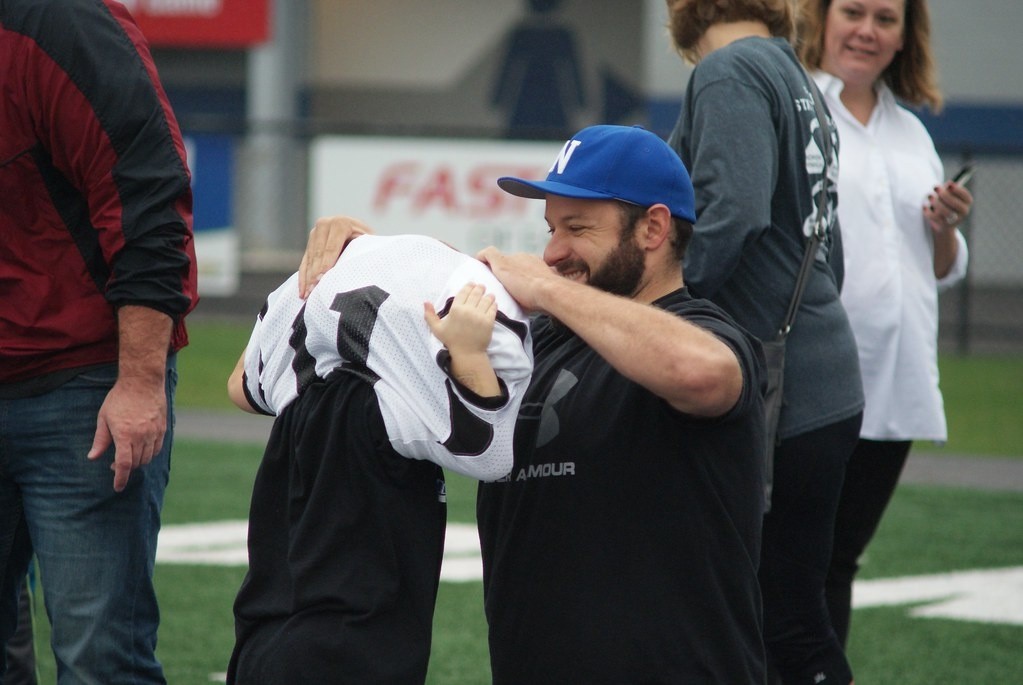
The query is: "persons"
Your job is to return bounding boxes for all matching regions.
[296,124,770,685]
[794,0,976,647]
[666,0,865,684]
[1,2,202,683]
[224,233,534,685]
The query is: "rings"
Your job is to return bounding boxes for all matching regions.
[947,214,958,224]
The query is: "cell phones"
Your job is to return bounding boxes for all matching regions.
[952,162,978,186]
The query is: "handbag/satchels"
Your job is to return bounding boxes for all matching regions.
[760,329,787,517]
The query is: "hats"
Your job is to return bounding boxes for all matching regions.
[497,124,696,223]
